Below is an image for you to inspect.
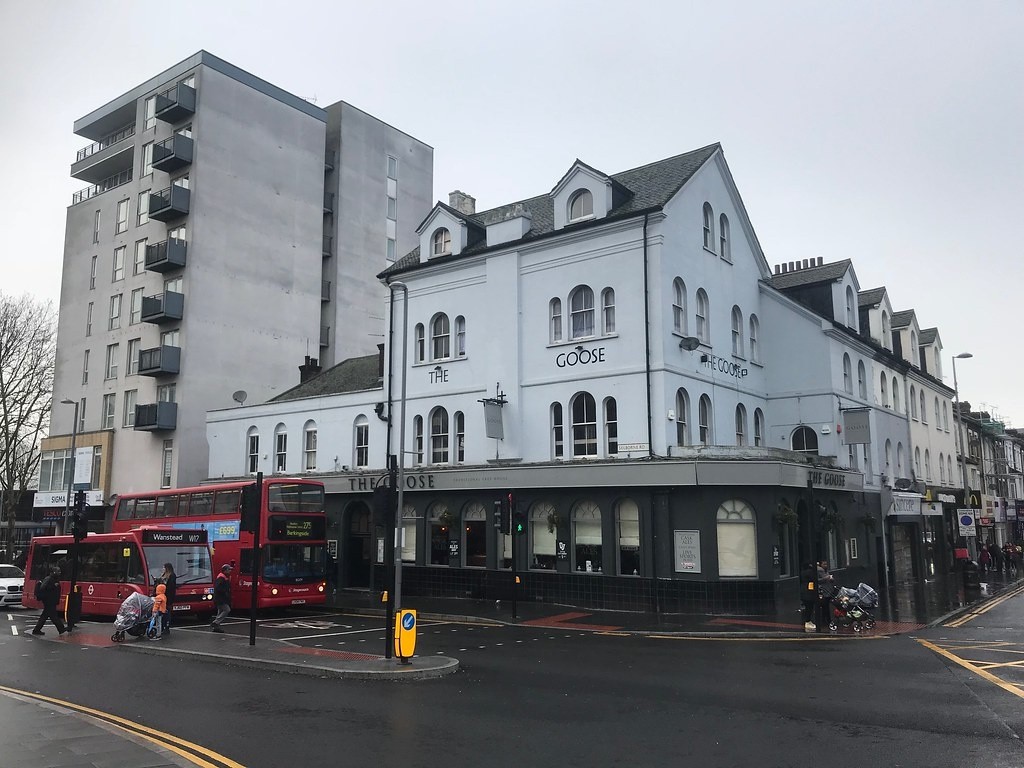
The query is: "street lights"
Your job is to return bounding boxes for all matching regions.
[59,397,79,535]
[951,353,979,570]
[388,280,409,609]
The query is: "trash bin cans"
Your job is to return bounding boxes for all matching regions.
[964,560,979,589]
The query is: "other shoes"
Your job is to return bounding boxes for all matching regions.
[59,628,72,635]
[32,629,45,635]
[162,630,170,634]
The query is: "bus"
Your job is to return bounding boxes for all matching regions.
[22,527,217,623]
[113,478,329,612]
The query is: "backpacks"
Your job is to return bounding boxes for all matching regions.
[35,580,43,601]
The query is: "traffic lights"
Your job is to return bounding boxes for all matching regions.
[72,509,87,541]
[513,510,525,537]
[494,498,510,535]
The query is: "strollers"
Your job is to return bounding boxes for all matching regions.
[819,583,879,633]
[111,593,157,642]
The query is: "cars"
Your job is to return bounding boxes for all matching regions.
[0,562,26,607]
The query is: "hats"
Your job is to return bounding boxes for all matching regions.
[222,564,233,570]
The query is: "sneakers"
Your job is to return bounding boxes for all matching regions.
[211,622,224,633]
[150,636,162,641]
[804,621,816,629]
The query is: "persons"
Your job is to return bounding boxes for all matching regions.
[210,563,234,634]
[29,567,69,636]
[151,563,177,635]
[815,560,835,629]
[800,558,817,629]
[976,534,1024,574]
[150,584,168,641]
